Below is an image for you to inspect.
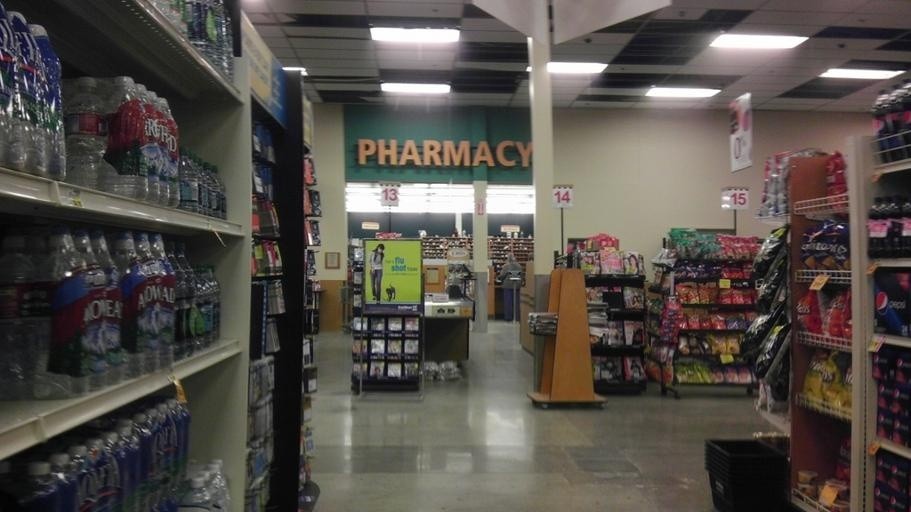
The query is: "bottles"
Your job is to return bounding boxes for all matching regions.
[1,395,234,511]
[872,77,911,162]
[0,1,232,222]
[313,280,321,291]
[0,225,222,393]
[422,360,455,386]
[876,291,908,336]
[864,194,911,259]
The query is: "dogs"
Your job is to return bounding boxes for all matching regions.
[386,282,396,302]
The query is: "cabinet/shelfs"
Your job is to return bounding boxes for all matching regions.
[239,87,307,512]
[574,267,648,397]
[785,196,862,512]
[488,237,535,287]
[644,250,767,397]
[417,236,474,260]
[0,0,257,512]
[752,209,794,436]
[303,179,325,400]
[850,152,910,512]
[527,262,609,411]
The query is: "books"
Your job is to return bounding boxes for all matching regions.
[527,314,556,334]
[578,250,647,383]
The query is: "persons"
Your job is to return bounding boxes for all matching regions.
[500,252,521,322]
[369,243,383,304]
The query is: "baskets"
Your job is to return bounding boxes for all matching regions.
[703,436,790,512]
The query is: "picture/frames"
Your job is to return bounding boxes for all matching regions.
[325,252,340,270]
